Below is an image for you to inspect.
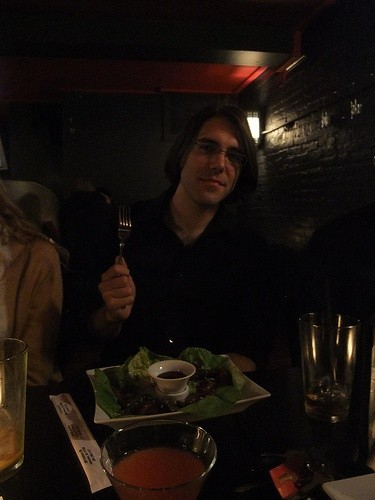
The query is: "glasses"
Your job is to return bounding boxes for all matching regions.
[193,138,248,166]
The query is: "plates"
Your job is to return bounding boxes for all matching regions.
[322,472,375,499]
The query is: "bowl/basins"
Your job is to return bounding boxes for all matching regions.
[98,420,217,499]
[86,354,271,432]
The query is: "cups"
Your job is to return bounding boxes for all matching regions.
[298,311,362,423]
[0,337,29,482]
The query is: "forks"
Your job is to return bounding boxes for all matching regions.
[118,205,132,265]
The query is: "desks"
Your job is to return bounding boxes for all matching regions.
[1,363,375,500]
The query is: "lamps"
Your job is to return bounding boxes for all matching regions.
[245,109,264,145]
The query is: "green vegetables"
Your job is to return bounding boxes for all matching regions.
[94,347,252,418]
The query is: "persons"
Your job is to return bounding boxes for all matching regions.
[0,179,65,389]
[65,103,289,370]
[58,186,116,255]
